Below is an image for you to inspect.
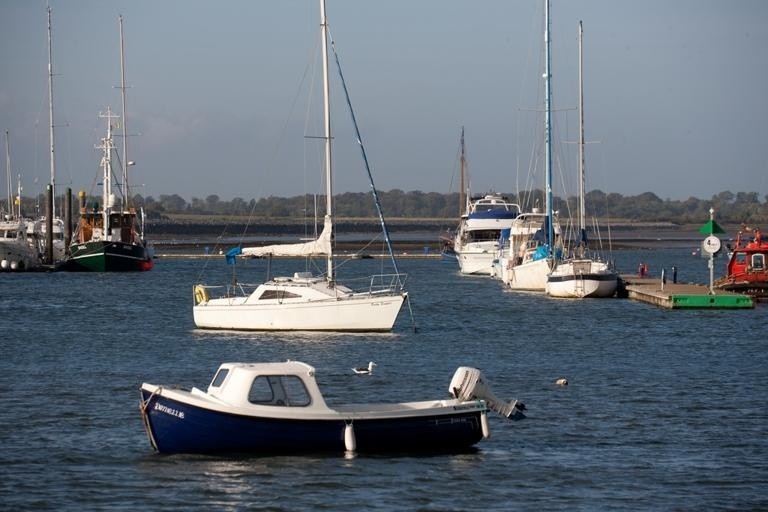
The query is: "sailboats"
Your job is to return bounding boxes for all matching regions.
[188,2,410,335]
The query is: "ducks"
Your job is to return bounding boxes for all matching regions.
[350,361,377,375]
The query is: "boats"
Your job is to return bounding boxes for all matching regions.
[138,356,525,460]
[714,224,768,300]
